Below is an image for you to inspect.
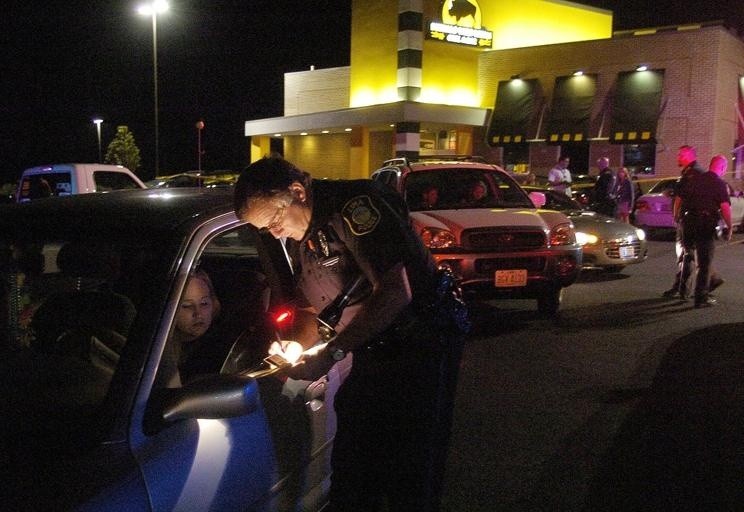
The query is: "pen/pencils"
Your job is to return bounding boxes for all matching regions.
[274,330,285,354]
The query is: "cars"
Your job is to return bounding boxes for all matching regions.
[144,169,237,188]
[496,186,651,286]
[546,184,642,223]
[630,175,744,236]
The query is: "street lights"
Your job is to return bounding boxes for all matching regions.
[195,120,205,174]
[91,118,103,160]
[134,1,170,176]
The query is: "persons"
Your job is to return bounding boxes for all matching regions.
[94,268,225,404]
[663,145,724,297]
[548,155,573,197]
[460,181,493,209]
[234,156,471,512]
[583,156,618,218]
[674,155,732,308]
[421,186,446,210]
[522,172,540,186]
[616,168,635,224]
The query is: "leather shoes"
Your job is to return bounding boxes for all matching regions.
[662,277,725,308]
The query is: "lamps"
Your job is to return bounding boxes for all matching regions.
[511,73,520,79]
[636,65,647,71]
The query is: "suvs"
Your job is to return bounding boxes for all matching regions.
[365,154,582,320]
[14,162,147,201]
[0,186,354,512]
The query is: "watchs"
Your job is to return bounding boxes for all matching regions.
[325,343,347,363]
